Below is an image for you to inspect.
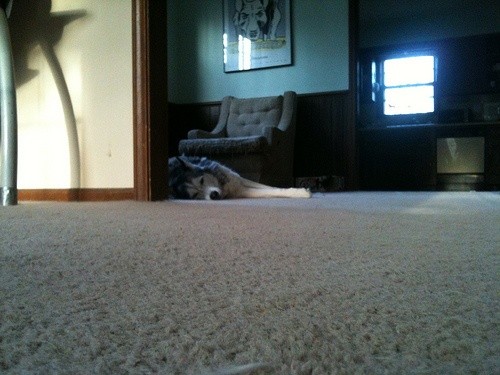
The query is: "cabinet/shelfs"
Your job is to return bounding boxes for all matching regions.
[359,122,500,192]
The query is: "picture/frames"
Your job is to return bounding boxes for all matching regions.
[222,0,292,73]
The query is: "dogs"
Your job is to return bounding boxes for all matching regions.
[168,155,313,201]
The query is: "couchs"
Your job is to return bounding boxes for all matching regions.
[179,91,299,188]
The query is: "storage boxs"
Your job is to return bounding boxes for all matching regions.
[298,175,345,193]
